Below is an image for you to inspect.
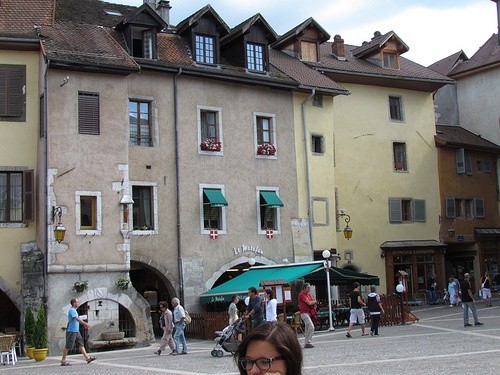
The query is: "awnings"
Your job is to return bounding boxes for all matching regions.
[304,265,381,287]
[199,258,327,303]
[203,190,228,207]
[261,192,283,208]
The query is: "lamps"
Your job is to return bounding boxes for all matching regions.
[336,209,353,240]
[52,206,67,245]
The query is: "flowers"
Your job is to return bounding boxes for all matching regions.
[200,139,222,151]
[257,141,276,155]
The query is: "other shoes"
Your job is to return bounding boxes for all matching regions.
[180,351,187,355]
[169,351,179,355]
[361,332,369,336]
[371,333,379,336]
[346,332,352,338]
[154,351,161,356]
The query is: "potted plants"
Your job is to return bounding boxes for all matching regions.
[115,279,131,290]
[23,303,36,360]
[72,279,88,292]
[32,304,49,362]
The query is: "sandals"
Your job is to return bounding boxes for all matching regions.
[60,361,72,366]
[86,357,97,363]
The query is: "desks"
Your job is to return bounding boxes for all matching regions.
[315,298,370,331]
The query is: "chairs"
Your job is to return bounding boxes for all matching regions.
[0,326,21,367]
[287,311,305,338]
[278,313,284,323]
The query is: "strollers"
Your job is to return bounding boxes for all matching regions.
[209,314,254,357]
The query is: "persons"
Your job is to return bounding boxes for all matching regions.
[264,289,277,321]
[60,298,97,367]
[367,285,384,336]
[228,295,242,342]
[460,272,484,328]
[298,283,318,349]
[345,282,369,339]
[479,272,493,308]
[154,302,177,356]
[167,297,188,355]
[234,320,304,375]
[416,271,460,306]
[242,286,264,330]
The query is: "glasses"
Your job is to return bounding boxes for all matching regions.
[238,355,284,371]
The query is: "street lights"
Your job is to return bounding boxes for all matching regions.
[320,249,336,332]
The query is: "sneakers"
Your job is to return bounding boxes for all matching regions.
[474,322,484,327]
[305,344,313,349]
[464,323,472,327]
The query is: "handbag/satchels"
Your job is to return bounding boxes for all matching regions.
[184,311,192,325]
